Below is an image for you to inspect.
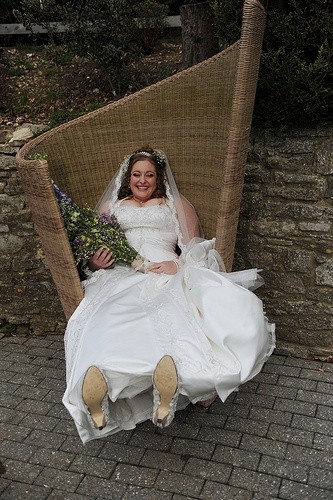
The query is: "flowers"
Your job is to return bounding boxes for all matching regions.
[27,152,152,276]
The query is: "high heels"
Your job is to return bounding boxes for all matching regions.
[81,365,109,431]
[151,355,179,424]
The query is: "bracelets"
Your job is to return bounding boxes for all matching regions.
[174,261,181,273]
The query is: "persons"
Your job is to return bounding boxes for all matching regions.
[64,146,218,431]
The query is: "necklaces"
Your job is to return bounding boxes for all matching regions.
[132,196,156,204]
